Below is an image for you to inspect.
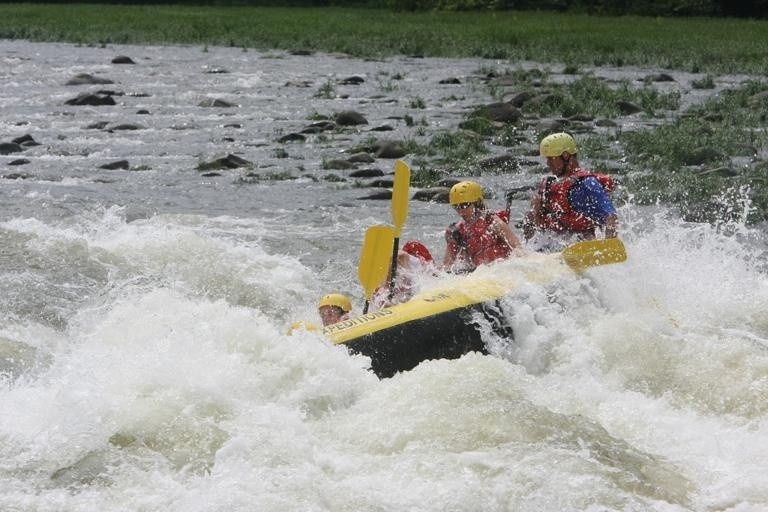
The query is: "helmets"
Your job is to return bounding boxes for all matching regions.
[448,181,483,206]
[317,293,352,313]
[539,132,578,157]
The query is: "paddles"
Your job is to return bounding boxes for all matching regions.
[359,225,394,314]
[391,162,410,299]
[555,237,627,272]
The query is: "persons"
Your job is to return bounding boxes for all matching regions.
[443,181,522,271]
[373,249,422,309]
[318,293,352,327]
[523,133,619,256]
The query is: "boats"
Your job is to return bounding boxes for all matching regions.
[286,250,585,380]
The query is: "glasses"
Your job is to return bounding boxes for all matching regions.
[451,202,470,209]
